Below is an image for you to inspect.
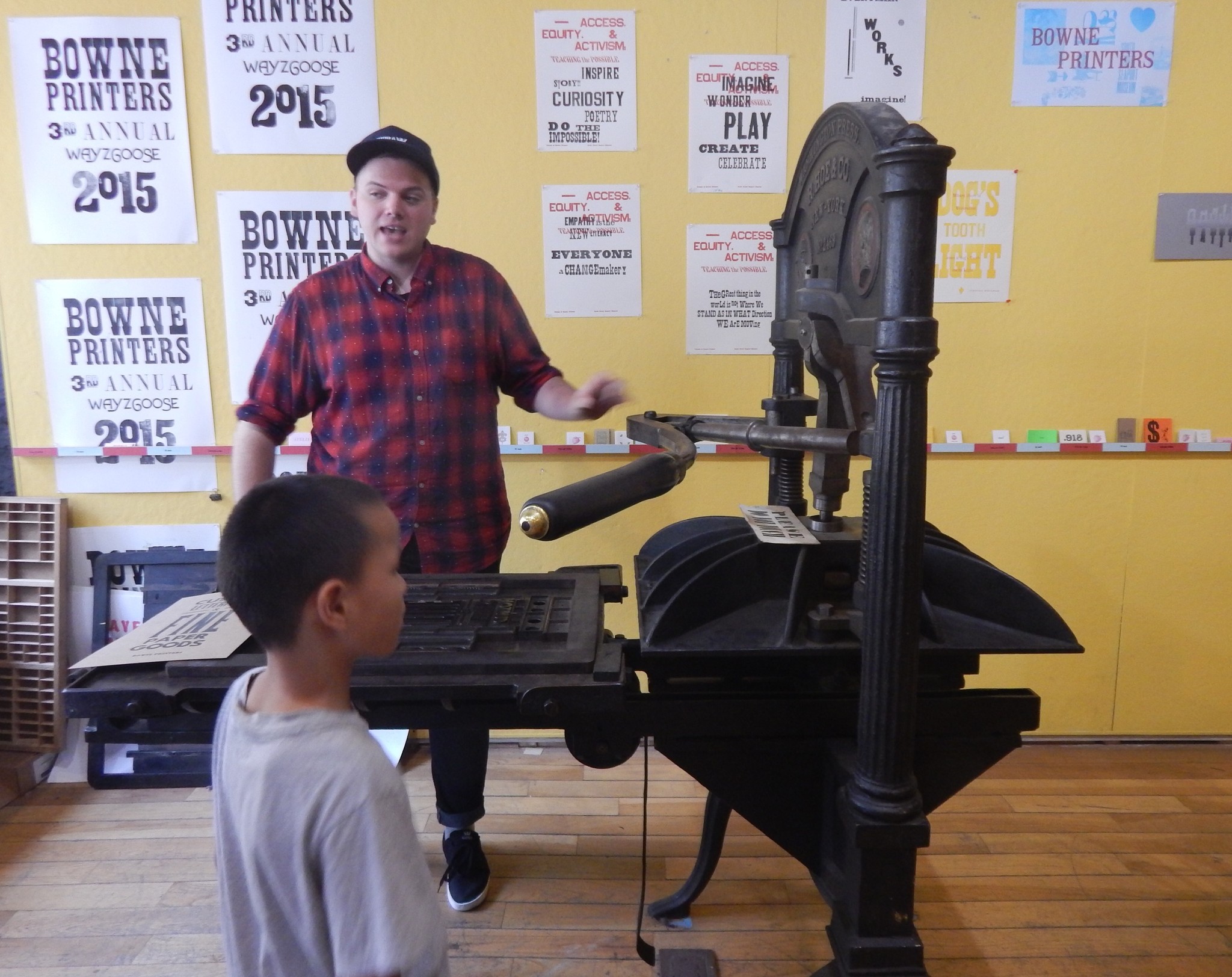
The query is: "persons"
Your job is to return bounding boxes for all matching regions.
[211,475,457,975]
[232,124,636,910]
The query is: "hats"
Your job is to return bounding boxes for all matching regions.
[347,124,439,192]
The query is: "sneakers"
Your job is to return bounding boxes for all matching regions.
[436,830,490,911]
